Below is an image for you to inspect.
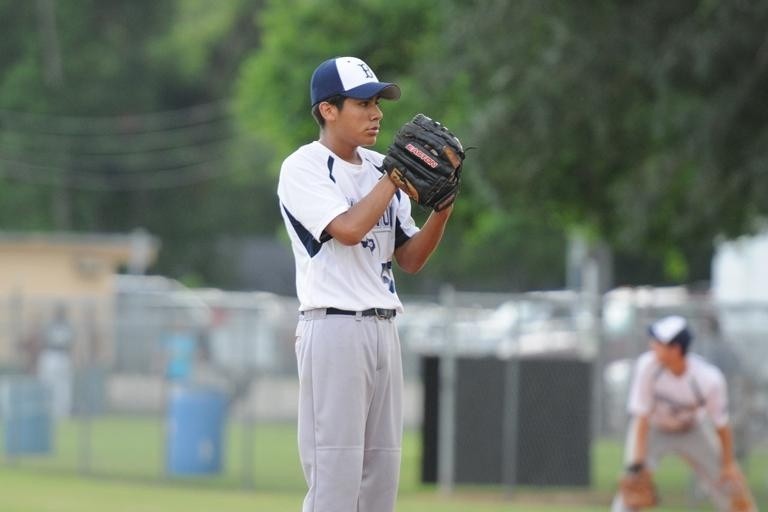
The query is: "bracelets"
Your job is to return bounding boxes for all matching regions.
[625,463,642,473]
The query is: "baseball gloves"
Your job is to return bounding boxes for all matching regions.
[621,463,659,508]
[381,113,466,210]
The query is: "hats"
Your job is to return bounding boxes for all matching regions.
[646,315,691,351]
[310,56,400,107]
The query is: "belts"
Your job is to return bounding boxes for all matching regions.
[301,307,397,320]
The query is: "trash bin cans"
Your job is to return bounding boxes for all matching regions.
[5,381,51,453]
[165,388,222,474]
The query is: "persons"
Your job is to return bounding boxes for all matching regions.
[274,54,461,511]
[609,314,758,512]
